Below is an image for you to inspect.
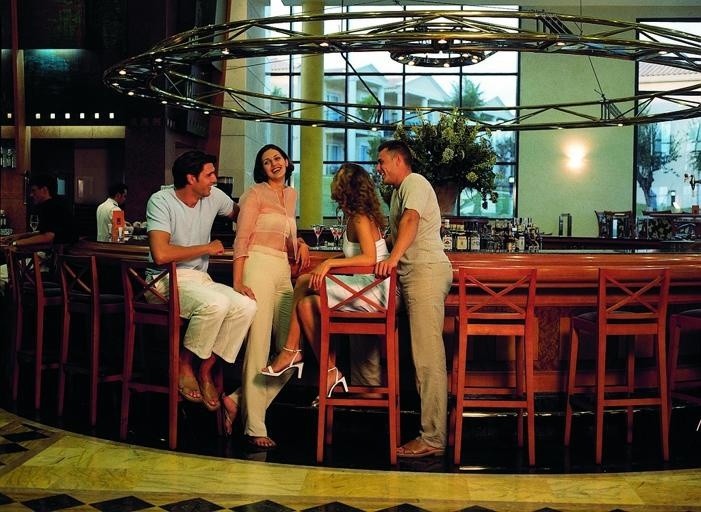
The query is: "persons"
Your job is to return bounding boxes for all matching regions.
[232,143,311,449]
[345,140,454,458]
[259,161,393,408]
[28,174,80,255]
[141,151,257,412]
[95,183,132,244]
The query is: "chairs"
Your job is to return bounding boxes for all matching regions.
[447,265,539,466]
[7,251,59,410]
[118,259,230,450]
[56,255,124,426]
[315,265,401,463]
[558,270,674,465]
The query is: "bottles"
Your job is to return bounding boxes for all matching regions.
[5,149,13,168]
[0,213,6,229]
[609,217,619,240]
[647,189,657,211]
[439,214,543,253]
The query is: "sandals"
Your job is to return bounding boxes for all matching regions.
[396,435,443,461]
[312,368,349,408]
[259,347,306,380]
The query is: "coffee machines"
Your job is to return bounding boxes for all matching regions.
[214,175,239,234]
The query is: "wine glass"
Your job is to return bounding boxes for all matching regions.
[312,224,324,249]
[29,214,38,233]
[329,225,345,250]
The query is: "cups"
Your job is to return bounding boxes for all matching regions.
[106,223,112,234]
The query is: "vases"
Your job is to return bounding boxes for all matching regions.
[371,99,503,216]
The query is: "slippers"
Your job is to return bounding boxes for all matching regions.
[220,392,232,439]
[244,437,277,451]
[177,376,202,404]
[200,381,220,412]
